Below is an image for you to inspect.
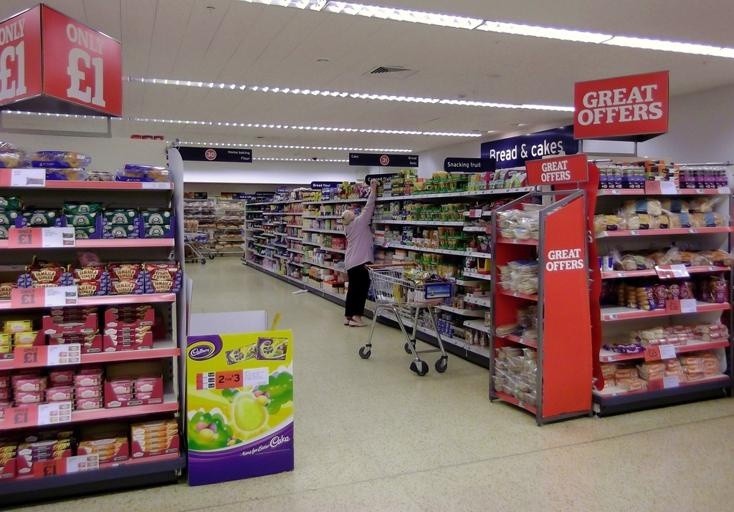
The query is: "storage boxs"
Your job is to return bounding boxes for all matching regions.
[186,275,294,486]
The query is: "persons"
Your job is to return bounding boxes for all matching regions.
[340,178,377,328]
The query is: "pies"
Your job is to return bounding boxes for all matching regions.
[616,282,649,310]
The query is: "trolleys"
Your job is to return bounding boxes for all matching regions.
[184,218,215,264]
[356,260,455,375]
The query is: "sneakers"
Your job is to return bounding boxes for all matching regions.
[344,314,368,327]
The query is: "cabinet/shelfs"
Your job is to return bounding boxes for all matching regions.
[552,160,733,417]
[0,147,188,497]
[183,197,246,257]
[239,183,541,360]
[488,149,594,428]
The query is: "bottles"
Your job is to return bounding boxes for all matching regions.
[599,164,648,190]
[678,164,728,189]
[463,232,489,349]
